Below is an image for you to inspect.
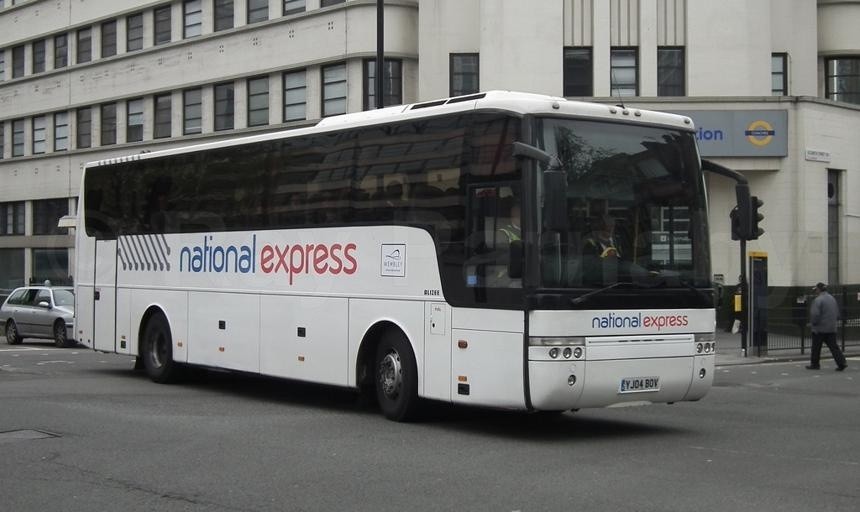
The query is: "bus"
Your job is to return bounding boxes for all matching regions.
[71,90,750,422]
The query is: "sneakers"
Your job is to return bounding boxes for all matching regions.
[804,364,849,372]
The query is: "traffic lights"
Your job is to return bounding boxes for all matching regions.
[753,196,765,240]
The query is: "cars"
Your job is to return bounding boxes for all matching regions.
[1,287,75,347]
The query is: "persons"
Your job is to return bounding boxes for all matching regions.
[146,180,413,230]
[723,275,747,334]
[485,201,522,288]
[805,281,849,372]
[582,214,623,284]
